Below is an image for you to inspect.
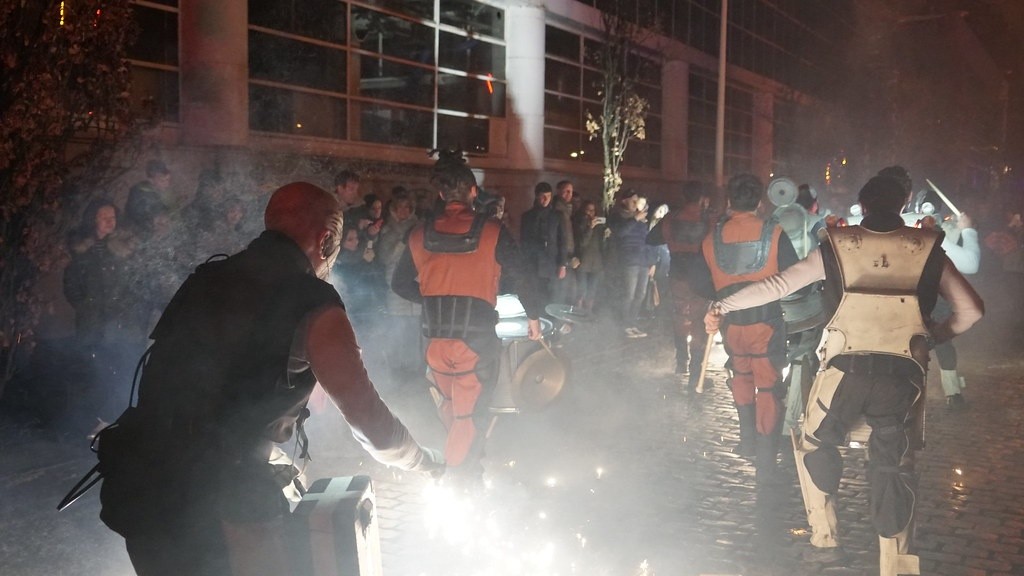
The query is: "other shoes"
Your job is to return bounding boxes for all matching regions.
[734,443,756,456]
[756,465,786,480]
[803,545,838,562]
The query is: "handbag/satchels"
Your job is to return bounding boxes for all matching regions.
[56,407,246,539]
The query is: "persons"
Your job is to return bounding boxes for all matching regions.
[332,173,436,374]
[391,164,541,496]
[0,160,277,441]
[703,169,985,576]
[473,169,674,339]
[641,177,976,486]
[125,183,449,576]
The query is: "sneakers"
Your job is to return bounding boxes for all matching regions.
[623,327,647,338]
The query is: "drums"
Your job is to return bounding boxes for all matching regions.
[493,292,528,320]
[487,316,557,414]
[780,280,828,336]
[845,391,927,451]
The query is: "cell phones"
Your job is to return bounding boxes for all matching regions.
[637,198,647,211]
[596,217,606,224]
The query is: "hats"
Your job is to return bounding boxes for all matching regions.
[617,186,639,200]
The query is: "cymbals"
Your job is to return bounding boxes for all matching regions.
[545,302,598,324]
[508,347,575,414]
